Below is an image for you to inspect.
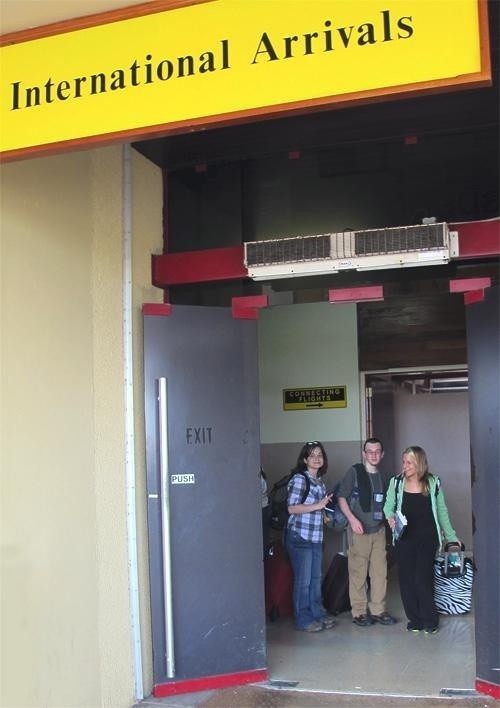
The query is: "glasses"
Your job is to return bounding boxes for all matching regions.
[307,441,320,446]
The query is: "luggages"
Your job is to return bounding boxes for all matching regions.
[321,528,351,615]
[265,538,293,622]
[432,542,473,616]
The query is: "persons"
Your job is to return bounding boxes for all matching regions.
[284,440,334,632]
[383,445,461,634]
[335,438,396,627]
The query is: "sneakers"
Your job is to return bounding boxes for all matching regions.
[295,618,336,633]
[352,614,440,634]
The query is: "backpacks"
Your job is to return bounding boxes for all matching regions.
[269,470,310,530]
[324,467,358,533]
